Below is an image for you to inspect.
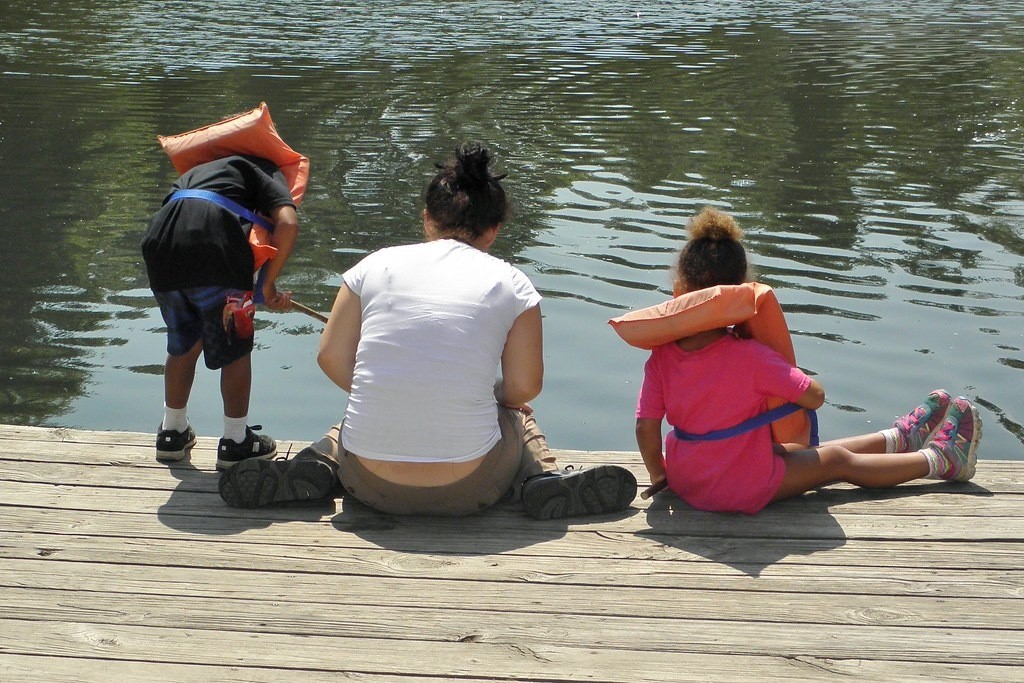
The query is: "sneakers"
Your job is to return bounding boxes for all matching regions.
[522,465,637,520]
[893,389,983,482]
[217,424,277,470]
[217,444,336,508]
[155,416,197,461]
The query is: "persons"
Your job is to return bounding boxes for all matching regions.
[634,204,984,512]
[142,99,313,464]
[217,144,639,523]
